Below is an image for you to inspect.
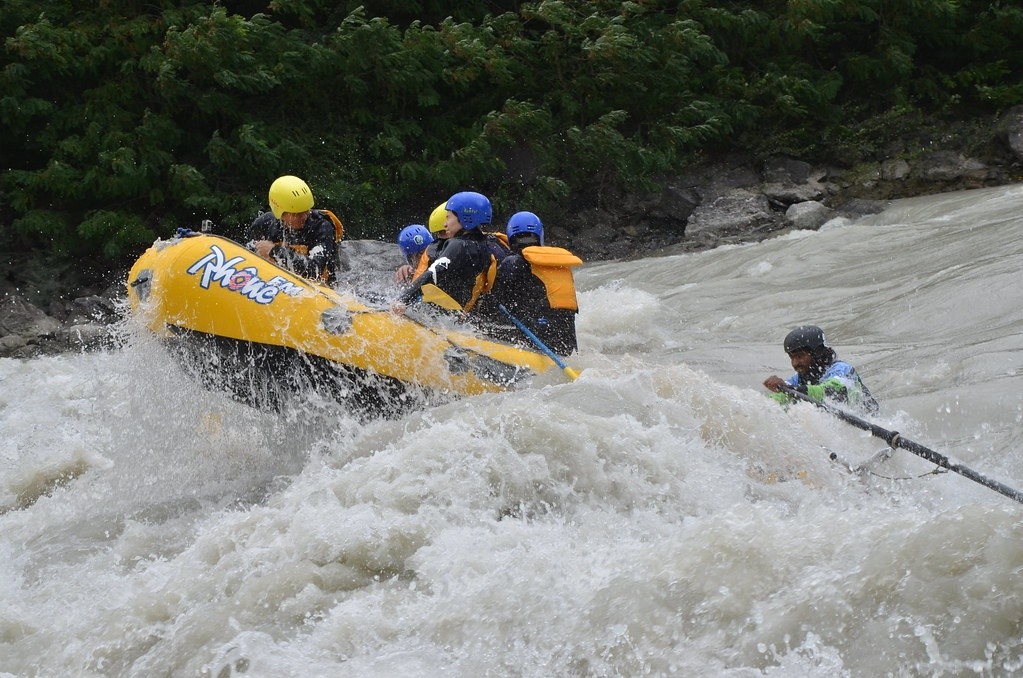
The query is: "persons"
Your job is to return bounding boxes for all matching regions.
[762,324,879,420]
[245,174,344,290]
[392,191,583,358]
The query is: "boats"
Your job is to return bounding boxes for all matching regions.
[130,223,582,421]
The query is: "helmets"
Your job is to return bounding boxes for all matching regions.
[429,201,448,233]
[445,191,492,229]
[506,211,544,246]
[269,175,314,219]
[783,326,825,354]
[398,224,434,255]
